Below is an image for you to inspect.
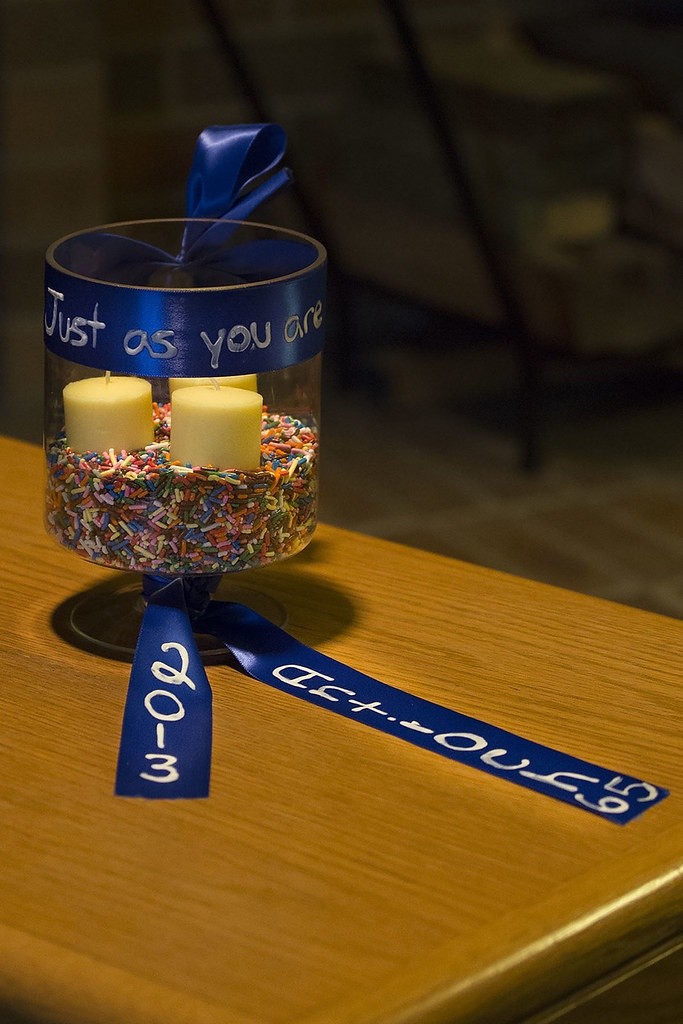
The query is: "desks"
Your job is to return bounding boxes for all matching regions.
[0,436,683,1024]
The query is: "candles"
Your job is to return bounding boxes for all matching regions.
[169,378,263,471]
[62,371,154,455]
[169,373,258,401]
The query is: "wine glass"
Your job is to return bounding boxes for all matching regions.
[43,219,327,663]
